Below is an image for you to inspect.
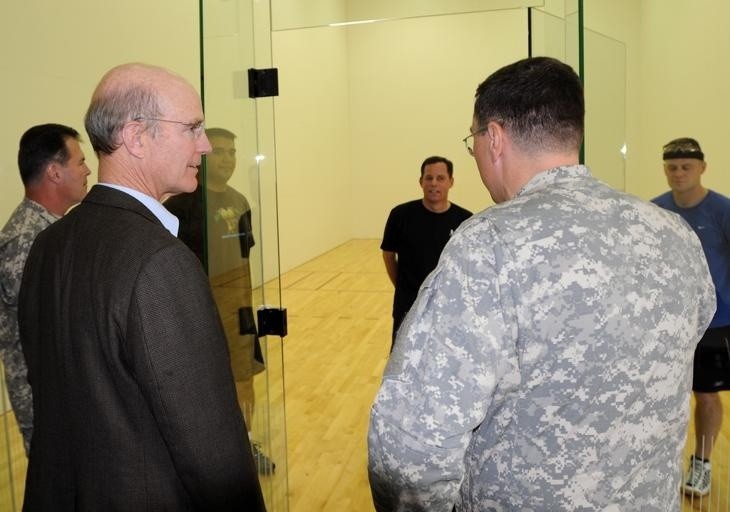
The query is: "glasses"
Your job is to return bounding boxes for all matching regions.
[134,116,206,141]
[463,117,504,158]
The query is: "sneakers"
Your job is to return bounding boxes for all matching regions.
[250,444,276,473]
[681,455,712,498]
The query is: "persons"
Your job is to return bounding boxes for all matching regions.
[164,127,276,476]
[368,56,719,511]
[17,62,271,511]
[0,124,92,458]
[380,156,472,351]
[650,137,730,497]
